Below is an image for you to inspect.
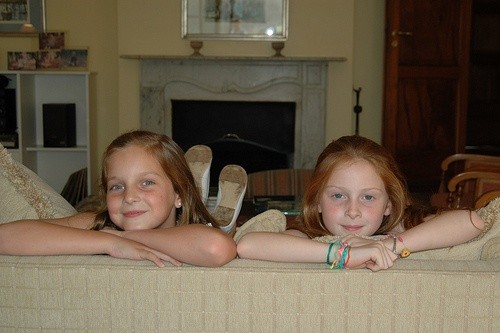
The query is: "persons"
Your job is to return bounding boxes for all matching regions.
[0,131,248,268]
[232,135,485,272]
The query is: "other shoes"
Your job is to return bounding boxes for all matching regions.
[212,165,247,231]
[184,145,212,206]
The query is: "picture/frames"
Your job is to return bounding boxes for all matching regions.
[0,0,46,37]
[181,0,289,42]
[7,30,89,70]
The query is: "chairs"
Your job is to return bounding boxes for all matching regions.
[244,168,317,213]
[437,153,500,214]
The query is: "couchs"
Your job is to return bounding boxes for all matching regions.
[0,255,499,333]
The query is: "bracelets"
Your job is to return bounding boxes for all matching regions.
[382,233,411,258]
[327,241,351,271]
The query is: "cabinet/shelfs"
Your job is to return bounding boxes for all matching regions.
[383,1,500,201]
[0,68,97,213]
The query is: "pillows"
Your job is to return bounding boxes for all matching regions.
[311,194,499,260]
[0,144,78,226]
